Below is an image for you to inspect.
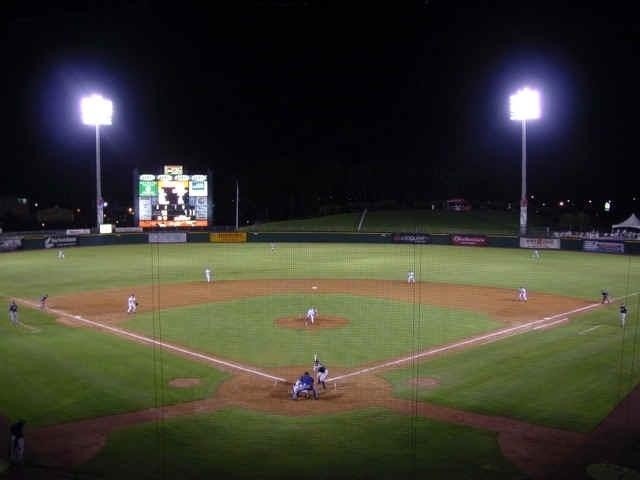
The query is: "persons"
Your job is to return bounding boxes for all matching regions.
[408,271,415,283]
[10,419,25,466]
[550,228,640,242]
[314,359,329,390]
[306,305,317,324]
[8,298,18,324]
[600,287,610,304]
[205,267,211,282]
[619,303,628,327]
[40,293,48,311]
[57,248,64,264]
[292,372,319,400]
[270,242,277,253]
[127,294,138,314]
[519,286,527,303]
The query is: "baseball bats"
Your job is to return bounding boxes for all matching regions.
[314,351,317,364]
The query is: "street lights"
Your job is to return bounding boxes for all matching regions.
[81,95,113,225]
[508,86,541,238]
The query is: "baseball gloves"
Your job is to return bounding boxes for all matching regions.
[136,302,138,305]
